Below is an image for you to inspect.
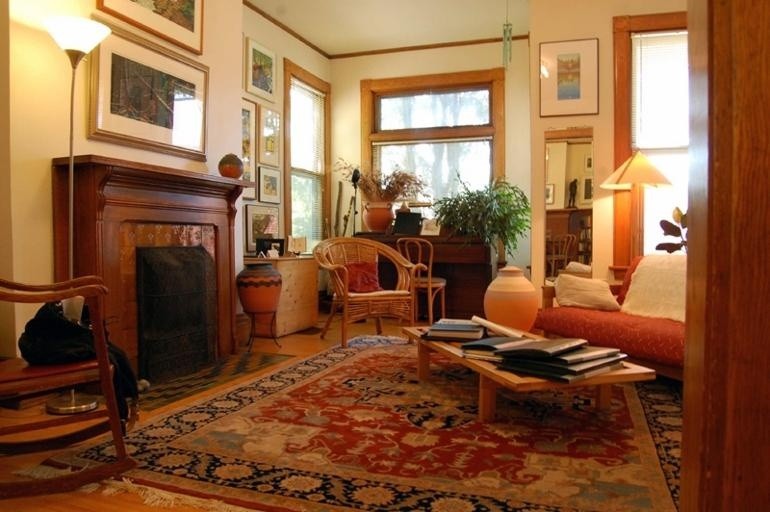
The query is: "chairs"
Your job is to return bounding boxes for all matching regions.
[0,275,140,498]
[392,238,448,329]
[547,226,592,276]
[313,235,430,350]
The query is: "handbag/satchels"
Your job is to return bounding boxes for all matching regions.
[18,302,109,366]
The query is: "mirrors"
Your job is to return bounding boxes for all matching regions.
[545,127,594,282]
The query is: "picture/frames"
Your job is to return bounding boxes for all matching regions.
[96,0,205,55]
[545,184,554,202]
[84,11,211,163]
[242,34,283,252]
[578,176,593,206]
[539,36,598,119]
[584,155,593,171]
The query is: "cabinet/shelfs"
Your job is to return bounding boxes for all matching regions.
[547,210,592,274]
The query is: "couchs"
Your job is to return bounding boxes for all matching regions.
[537,256,688,380]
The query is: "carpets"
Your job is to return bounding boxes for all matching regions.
[18,334,684,511]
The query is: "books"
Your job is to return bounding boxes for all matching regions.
[392,212,442,236]
[577,215,592,265]
[421,314,629,384]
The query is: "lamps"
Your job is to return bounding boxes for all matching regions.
[44,16,112,415]
[601,150,673,261]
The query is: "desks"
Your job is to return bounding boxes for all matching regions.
[244,257,318,338]
[354,233,491,324]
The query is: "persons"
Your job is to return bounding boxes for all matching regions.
[569,179,577,207]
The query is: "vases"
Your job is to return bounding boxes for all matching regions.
[238,262,282,314]
[218,154,244,180]
[483,265,538,335]
[362,202,396,235]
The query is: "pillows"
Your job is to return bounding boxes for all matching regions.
[554,276,619,311]
[564,262,590,273]
